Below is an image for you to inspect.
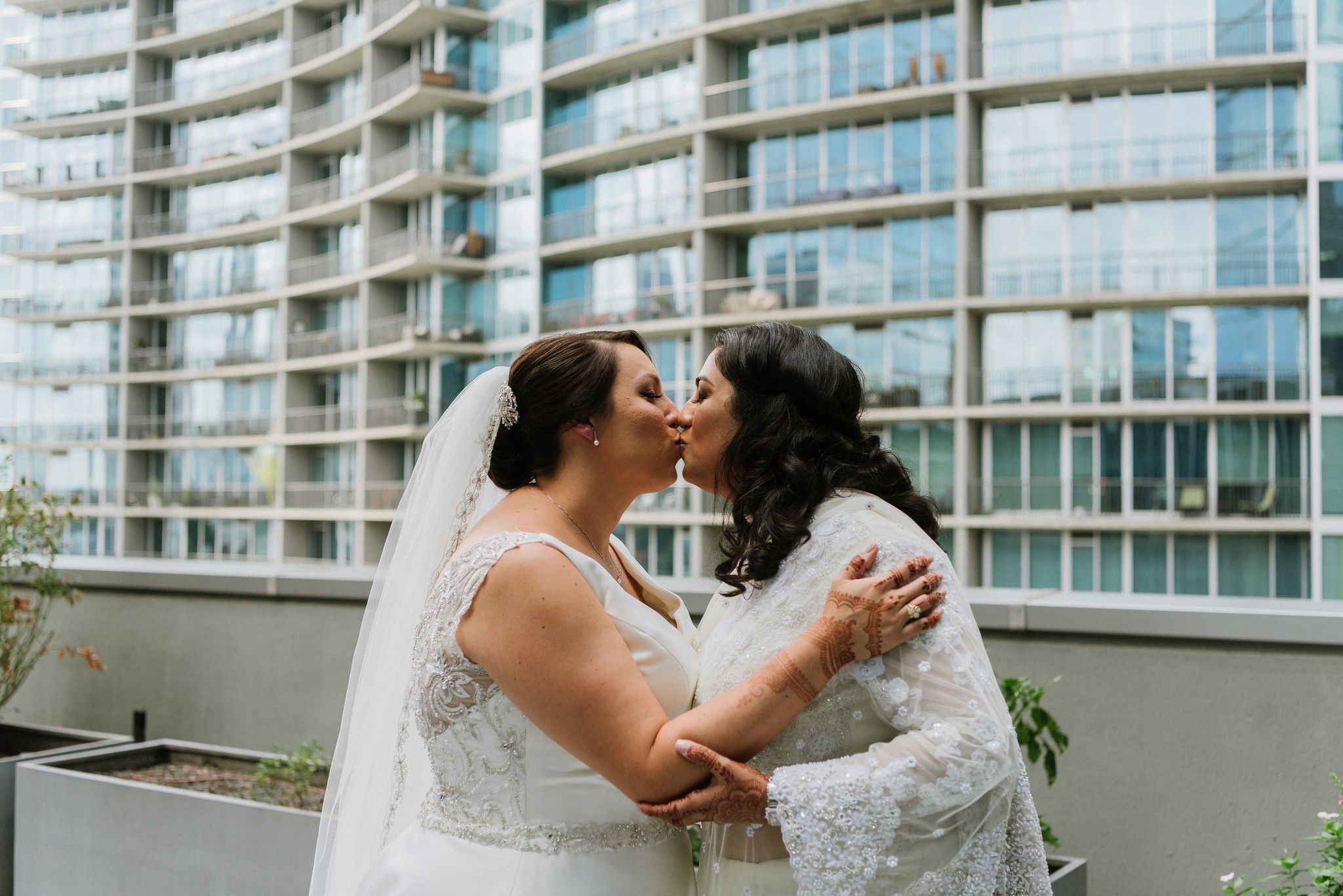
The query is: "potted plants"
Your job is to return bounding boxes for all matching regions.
[11,737,334,896]
[997,675,1083,896]
[1,473,147,896]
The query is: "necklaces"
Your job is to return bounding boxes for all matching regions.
[533,482,624,584]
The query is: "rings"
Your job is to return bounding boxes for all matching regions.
[905,602,924,620]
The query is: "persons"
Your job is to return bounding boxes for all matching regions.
[299,326,947,896]
[675,312,1057,896]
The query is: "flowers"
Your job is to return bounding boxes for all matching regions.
[1219,771,1343,896]
